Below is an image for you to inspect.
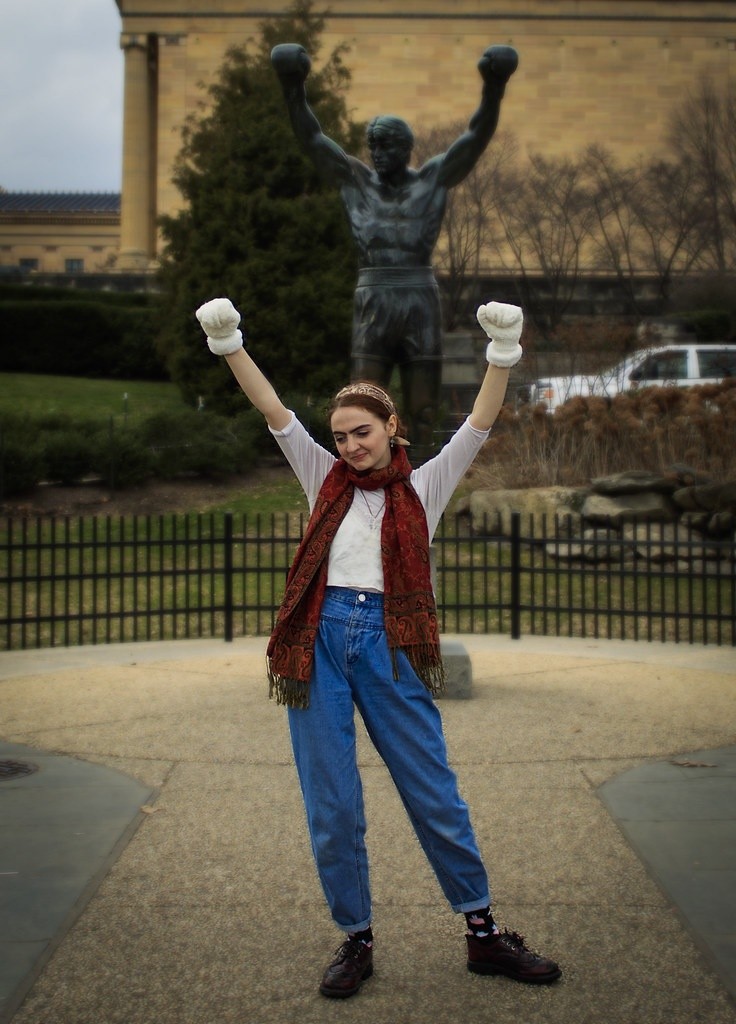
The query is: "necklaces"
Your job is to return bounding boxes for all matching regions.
[359,486,385,530]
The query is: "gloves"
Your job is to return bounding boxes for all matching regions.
[195,298,244,356]
[476,302,523,369]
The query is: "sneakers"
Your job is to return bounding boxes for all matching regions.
[319,940,375,998]
[464,926,562,985]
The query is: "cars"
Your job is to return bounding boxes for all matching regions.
[513,344,736,422]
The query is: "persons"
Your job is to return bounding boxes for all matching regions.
[195,297,562,1000]
[271,44,519,470]
